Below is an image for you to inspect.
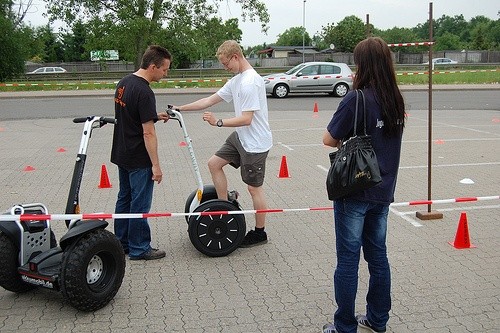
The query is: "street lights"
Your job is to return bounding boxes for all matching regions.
[303,0,307,63]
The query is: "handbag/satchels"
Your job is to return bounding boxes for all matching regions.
[327,88,382,200]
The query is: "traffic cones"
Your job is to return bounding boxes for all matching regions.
[447,211,477,250]
[97,164,113,189]
[276,155,292,179]
[313,101,319,112]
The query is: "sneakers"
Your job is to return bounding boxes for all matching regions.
[356,315,385,333]
[241,230,268,247]
[322,324,339,333]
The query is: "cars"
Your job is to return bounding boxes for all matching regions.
[421,57,458,64]
[26,66,69,74]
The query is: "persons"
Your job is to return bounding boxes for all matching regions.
[171,40,273,248]
[324,37,405,333]
[114,45,173,260]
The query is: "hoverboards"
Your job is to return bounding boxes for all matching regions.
[165,102,248,258]
[0,113,127,312]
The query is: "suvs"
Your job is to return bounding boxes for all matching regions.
[261,61,355,99]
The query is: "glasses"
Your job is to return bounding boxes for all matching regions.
[222,53,238,66]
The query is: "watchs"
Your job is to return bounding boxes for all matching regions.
[217,118,223,127]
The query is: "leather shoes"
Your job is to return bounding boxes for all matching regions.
[131,247,165,260]
